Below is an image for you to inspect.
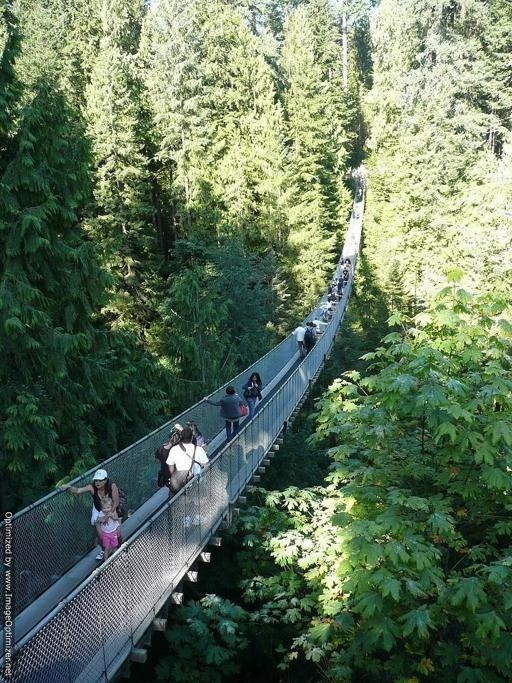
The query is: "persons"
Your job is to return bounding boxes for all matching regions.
[153,422,184,488]
[184,422,207,467]
[290,257,352,358]
[242,371,262,419]
[59,469,123,562]
[168,427,210,526]
[96,496,120,562]
[203,385,245,443]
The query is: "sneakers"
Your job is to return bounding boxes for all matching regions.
[224,440,228,446]
[182,514,192,528]
[95,550,105,559]
[193,514,208,525]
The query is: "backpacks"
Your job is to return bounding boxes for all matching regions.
[304,328,313,344]
[180,443,206,497]
[108,482,128,519]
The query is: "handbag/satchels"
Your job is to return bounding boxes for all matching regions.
[238,402,248,416]
[157,471,165,487]
[170,470,194,495]
[243,387,258,397]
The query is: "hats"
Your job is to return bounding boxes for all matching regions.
[92,469,108,480]
